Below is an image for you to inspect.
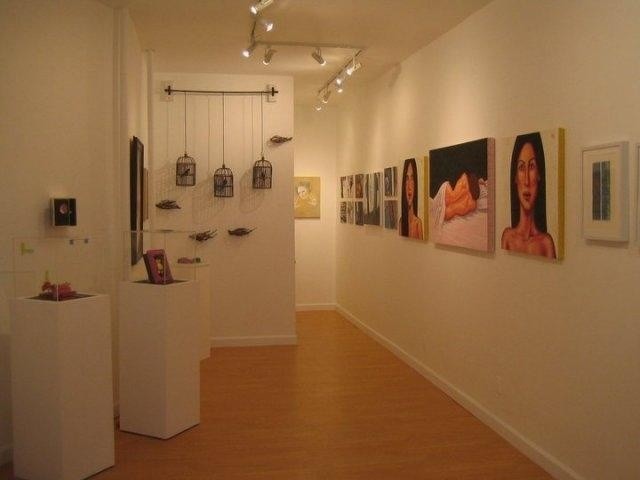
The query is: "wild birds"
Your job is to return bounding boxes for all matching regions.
[181,168,190,177]
[260,172,266,181]
[219,175,228,192]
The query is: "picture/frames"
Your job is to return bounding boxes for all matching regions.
[578,139,629,242]
[133,135,143,267]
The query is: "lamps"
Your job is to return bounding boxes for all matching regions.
[240,1,324,70]
[313,51,366,112]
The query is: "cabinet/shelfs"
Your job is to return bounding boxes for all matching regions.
[13,234,116,479]
[118,231,202,442]
[164,227,212,362]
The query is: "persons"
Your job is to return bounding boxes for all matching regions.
[398,158,425,240]
[500,131,557,261]
[441,169,481,225]
